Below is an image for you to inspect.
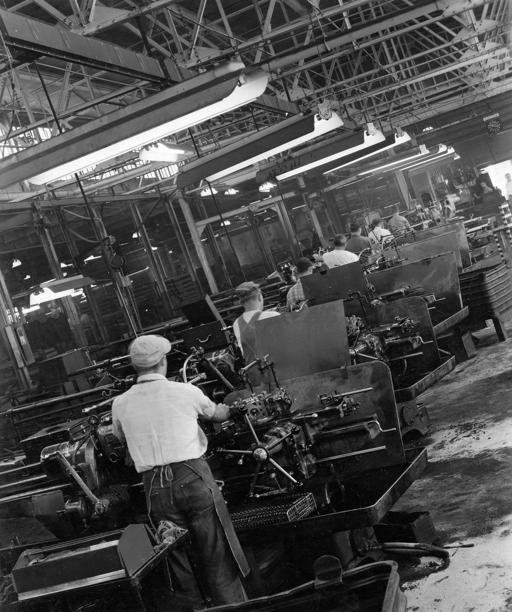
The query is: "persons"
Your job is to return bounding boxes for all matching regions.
[472,180,493,200]
[110,333,258,611]
[322,233,359,270]
[366,217,395,247]
[344,221,371,256]
[505,173,511,209]
[386,204,415,239]
[231,280,281,361]
[285,257,315,309]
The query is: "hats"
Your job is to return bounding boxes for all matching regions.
[234,281,261,301]
[350,221,362,232]
[128,332,173,369]
[371,205,399,226]
[296,258,314,271]
